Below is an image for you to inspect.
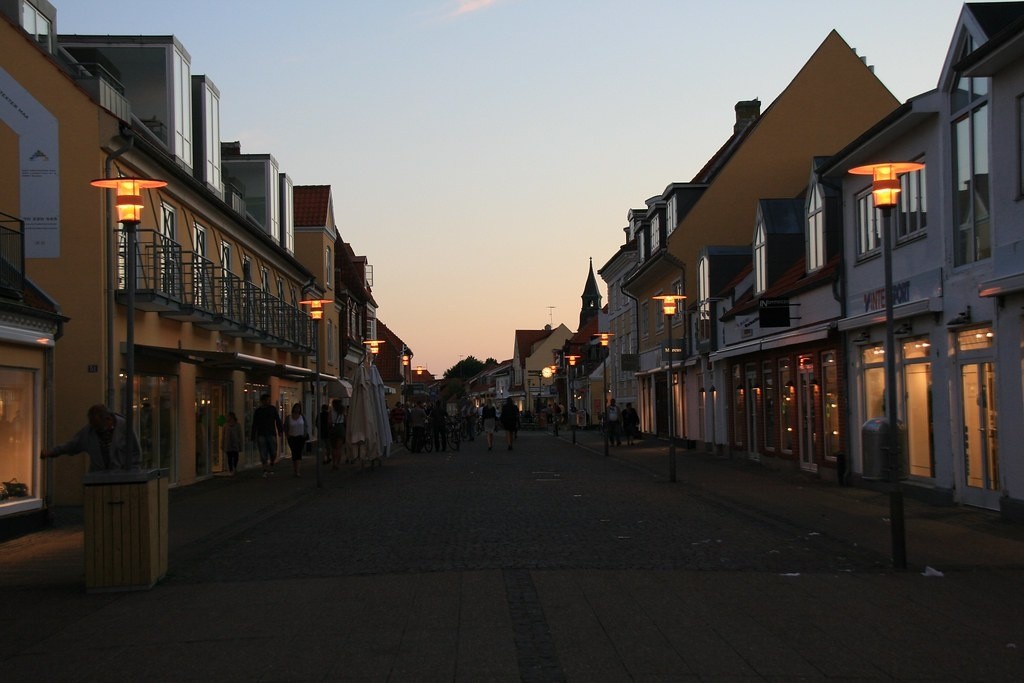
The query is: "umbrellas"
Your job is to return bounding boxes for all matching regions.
[346,365,393,468]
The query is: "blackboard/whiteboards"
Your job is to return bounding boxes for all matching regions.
[577,410,586,426]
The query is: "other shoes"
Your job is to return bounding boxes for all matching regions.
[611,444,614,448]
[617,442,621,447]
[508,445,512,450]
[297,473,300,477]
[435,448,446,452]
[411,449,422,454]
[487,447,493,452]
[628,443,635,447]
[229,472,234,478]
[263,470,275,480]
[332,465,339,471]
[468,437,475,442]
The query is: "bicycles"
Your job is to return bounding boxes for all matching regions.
[403,416,485,453]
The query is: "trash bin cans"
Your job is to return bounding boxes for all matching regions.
[82,468,169,593]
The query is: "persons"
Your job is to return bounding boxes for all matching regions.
[316,399,346,470]
[195,412,206,473]
[606,399,639,449]
[458,396,533,451]
[220,412,244,474]
[39,402,144,476]
[250,393,283,480]
[390,399,451,454]
[544,400,589,430]
[284,404,307,475]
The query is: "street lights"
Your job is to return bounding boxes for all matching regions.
[594,332,615,456]
[564,355,582,444]
[363,341,386,364]
[847,160,926,571]
[300,299,334,486]
[652,293,688,482]
[549,364,560,437]
[89,176,168,469]
[417,365,423,403]
[402,354,409,406]
